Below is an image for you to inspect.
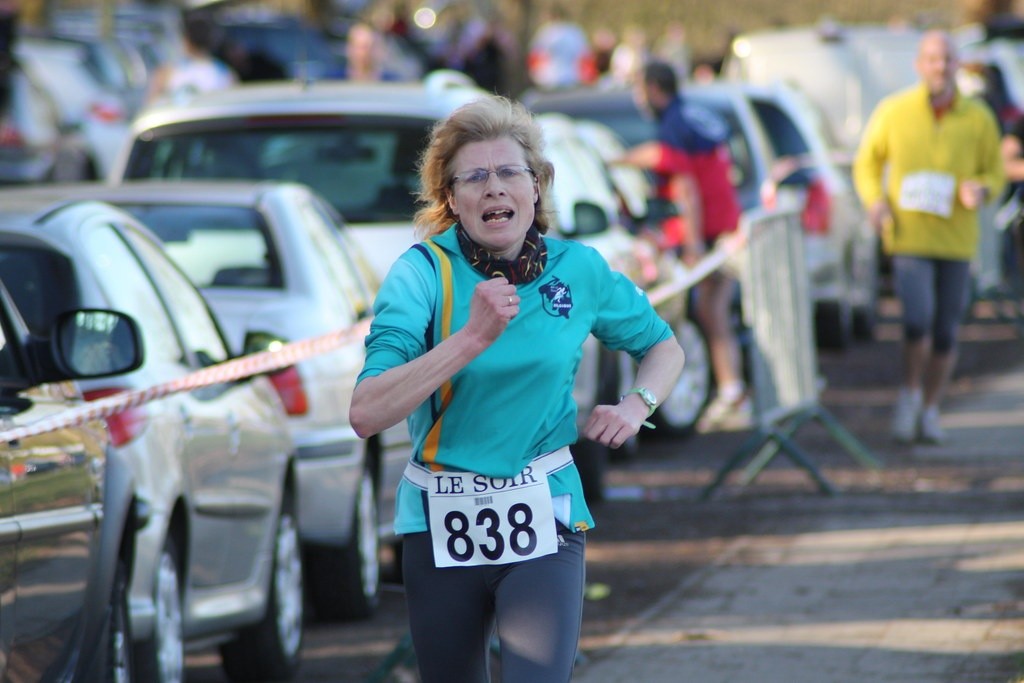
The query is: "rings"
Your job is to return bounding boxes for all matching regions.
[509,296,512,306]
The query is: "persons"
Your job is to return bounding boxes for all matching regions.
[852,27,1005,444]
[1000,116,1024,202]
[106,318,135,368]
[350,95,684,683]
[612,63,752,431]
[148,10,290,168]
[314,0,687,90]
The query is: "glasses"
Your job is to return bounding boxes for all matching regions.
[450,164,535,188]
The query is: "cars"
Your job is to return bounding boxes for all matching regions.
[0,0,1024,612]
[0,226,144,683]
[0,197,310,683]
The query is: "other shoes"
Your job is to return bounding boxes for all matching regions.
[697,396,759,433]
[889,389,942,444]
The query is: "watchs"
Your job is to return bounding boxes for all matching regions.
[621,387,657,429]
